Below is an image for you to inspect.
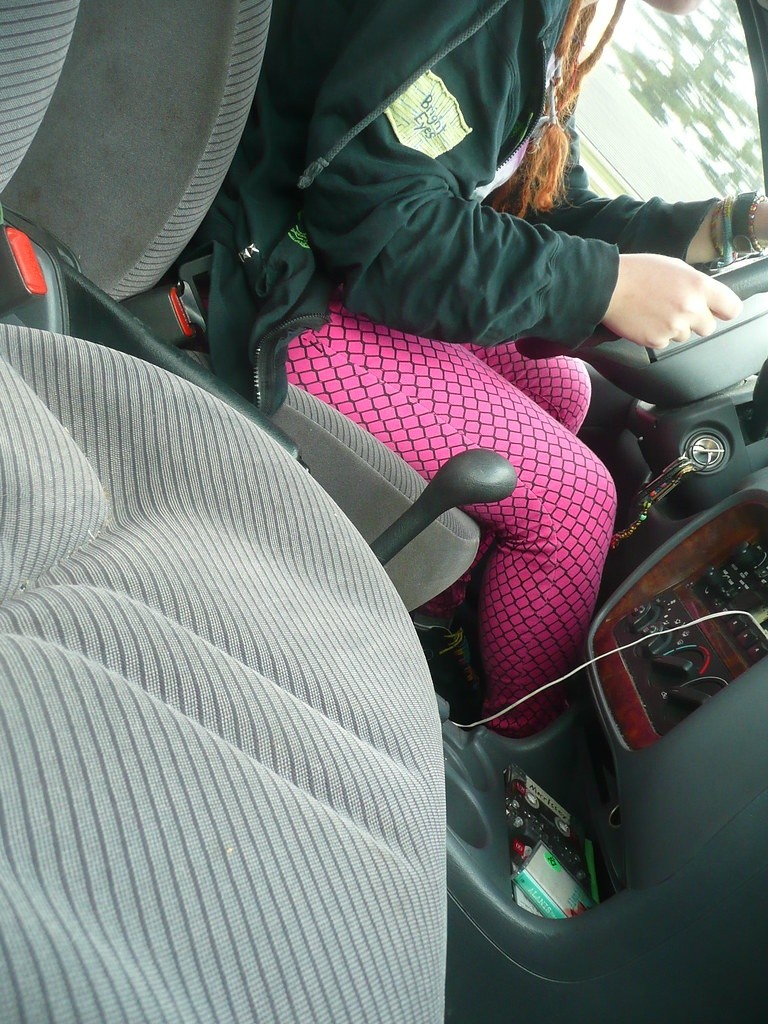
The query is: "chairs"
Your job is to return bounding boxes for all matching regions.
[0,1,480,612]
[0,0,447,1021]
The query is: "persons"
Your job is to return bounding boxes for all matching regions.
[182,0,768,742]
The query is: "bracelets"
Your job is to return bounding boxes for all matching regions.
[710,193,765,266]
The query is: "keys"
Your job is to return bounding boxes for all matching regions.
[635,448,726,508]
